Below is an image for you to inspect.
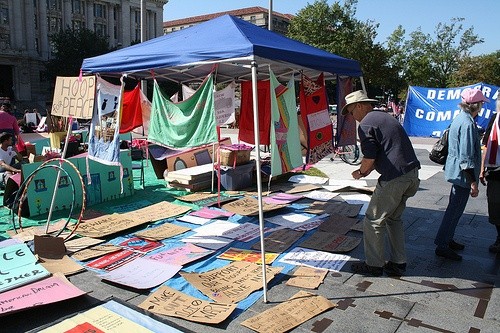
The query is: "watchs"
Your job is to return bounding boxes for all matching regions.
[357,169,362,175]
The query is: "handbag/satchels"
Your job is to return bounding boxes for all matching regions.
[429,127,451,165]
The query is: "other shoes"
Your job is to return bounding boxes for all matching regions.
[449,240,465,250]
[435,246,463,260]
[489,235,500,251]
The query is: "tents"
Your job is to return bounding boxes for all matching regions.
[45,14,373,304]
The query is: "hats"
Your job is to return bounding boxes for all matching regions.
[461,89,490,104]
[341,90,378,116]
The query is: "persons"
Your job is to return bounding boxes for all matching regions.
[339,90,420,277]
[0,100,21,146]
[479,90,497,254]
[435,86,485,262]
[24,109,41,128]
[0,132,30,187]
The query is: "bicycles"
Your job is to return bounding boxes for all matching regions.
[327,113,364,164]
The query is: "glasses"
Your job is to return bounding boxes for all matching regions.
[350,104,357,116]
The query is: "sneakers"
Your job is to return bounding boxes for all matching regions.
[384,260,406,275]
[351,261,383,277]
